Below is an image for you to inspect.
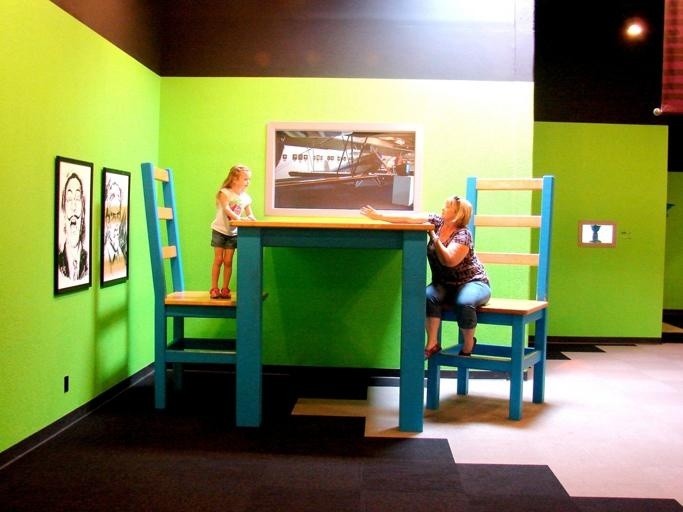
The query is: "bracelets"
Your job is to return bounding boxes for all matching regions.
[432,236,439,241]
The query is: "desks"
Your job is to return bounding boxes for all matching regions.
[228,220,436,433]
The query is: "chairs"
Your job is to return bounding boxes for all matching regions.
[141,162,269,409]
[426,176,554,420]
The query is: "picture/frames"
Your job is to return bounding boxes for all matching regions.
[577,220,616,247]
[265,122,424,216]
[54,155,94,293]
[100,166,130,288]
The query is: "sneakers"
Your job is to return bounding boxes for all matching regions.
[210,288,220,299]
[221,288,231,298]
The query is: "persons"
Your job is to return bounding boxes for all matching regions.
[55,171,87,280]
[209,164,259,298]
[357,195,490,360]
[102,177,127,282]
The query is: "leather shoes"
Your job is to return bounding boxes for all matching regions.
[425,342,442,359]
[459,337,477,355]
